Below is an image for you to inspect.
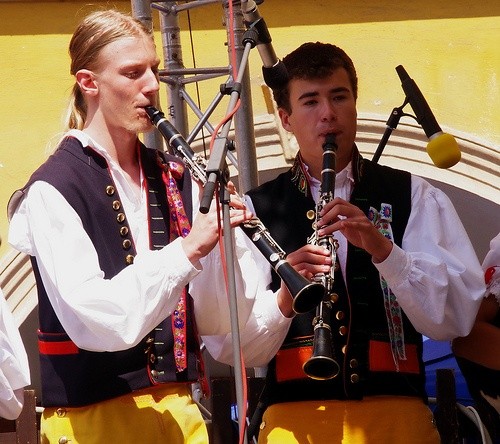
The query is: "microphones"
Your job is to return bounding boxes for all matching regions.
[241,0,290,89]
[396,65,461,169]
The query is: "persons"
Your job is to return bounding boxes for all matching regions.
[7,11,260,444]
[0,237,31,444]
[201,42,486,444]
